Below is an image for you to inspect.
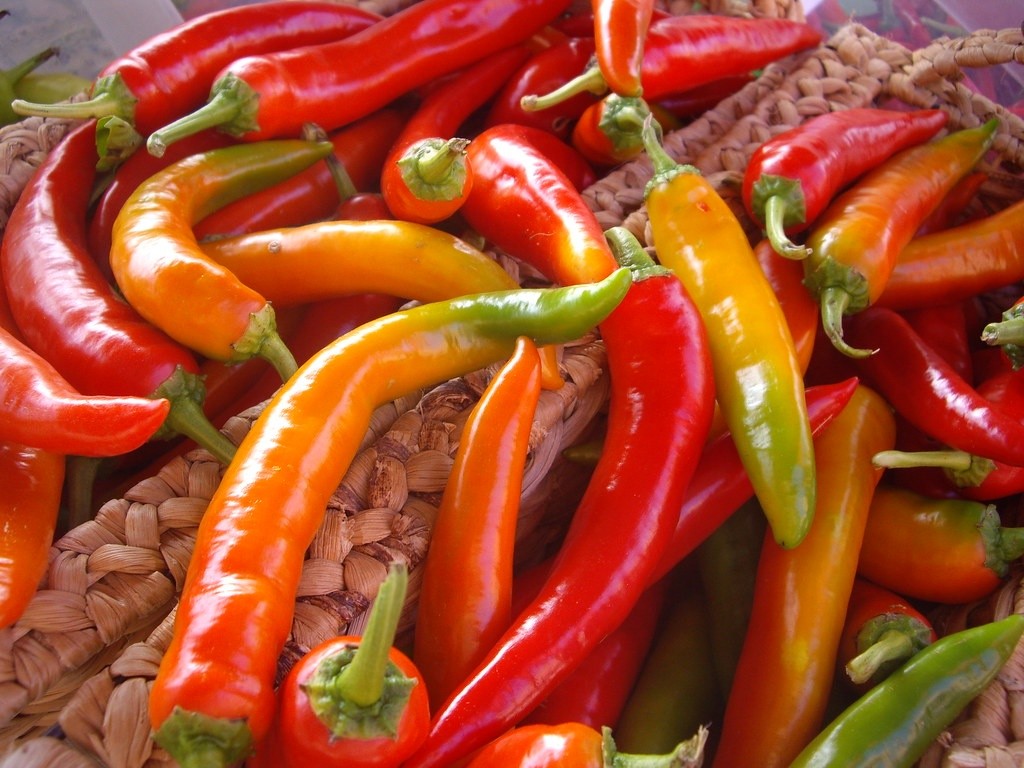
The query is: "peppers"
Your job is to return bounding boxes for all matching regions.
[0,0,1024,768]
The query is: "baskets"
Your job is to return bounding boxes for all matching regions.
[0,31,1024,768]
[0,0,815,768]
